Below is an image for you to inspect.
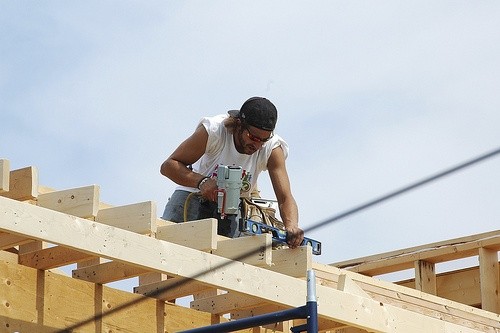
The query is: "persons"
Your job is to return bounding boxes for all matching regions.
[160,95,303,250]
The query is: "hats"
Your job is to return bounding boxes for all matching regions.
[228,97,277,131]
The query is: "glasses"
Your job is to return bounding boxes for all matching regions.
[245,126,274,142]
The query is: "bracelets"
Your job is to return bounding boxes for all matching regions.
[196,177,210,190]
